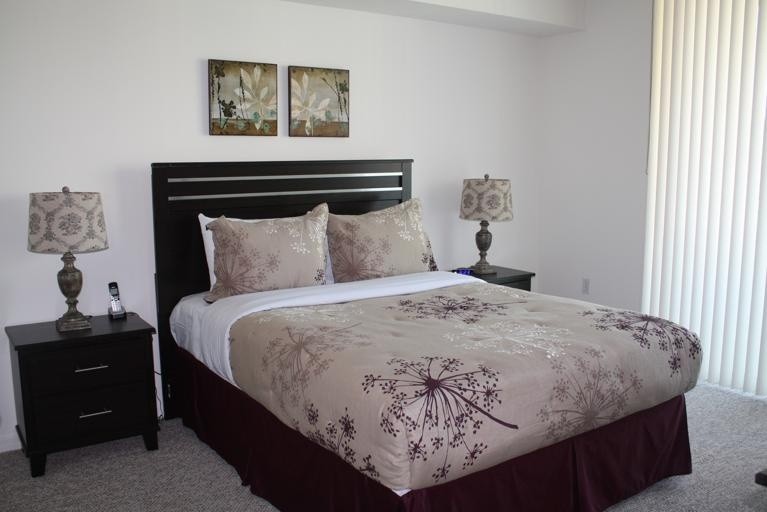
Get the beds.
[149,158,702,512]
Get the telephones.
[107,282,126,321]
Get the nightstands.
[449,263,535,292]
[4,311,157,478]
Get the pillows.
[197,197,439,304]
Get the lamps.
[458,173,514,275]
[26,186,108,333]
